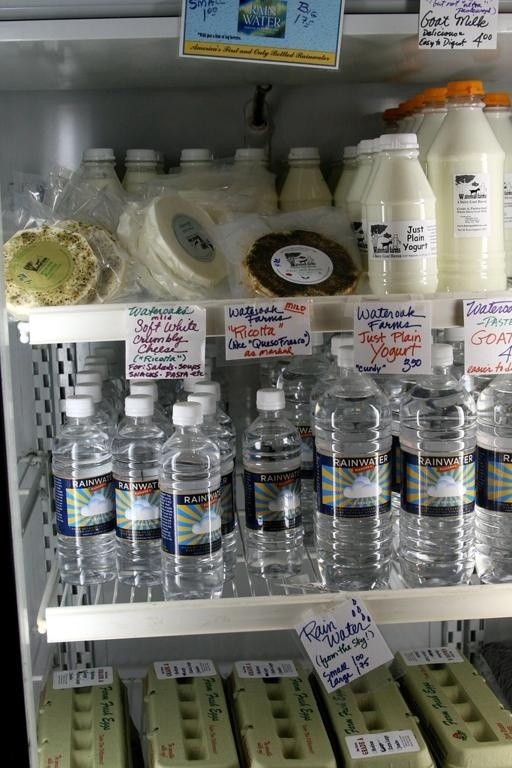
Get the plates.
[0,0,511,768]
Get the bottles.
[51,326,512,602]
[78,80,512,295]
